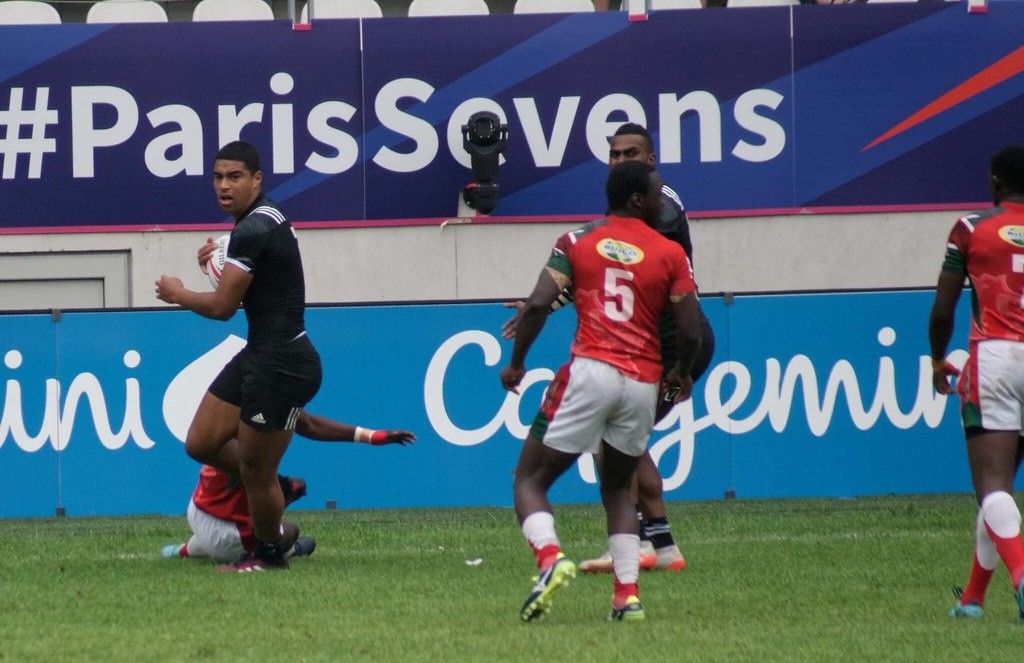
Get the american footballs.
[206,234,230,290]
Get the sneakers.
[603,595,645,621]
[520,551,576,623]
[1013,575,1024,624]
[647,545,685,572]
[162,543,177,557]
[579,541,658,574]
[283,478,305,508]
[218,544,289,573]
[282,536,315,560]
[947,601,984,617]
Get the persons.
[496,122,717,624]
[155,142,419,572]
[928,146,1024,626]
